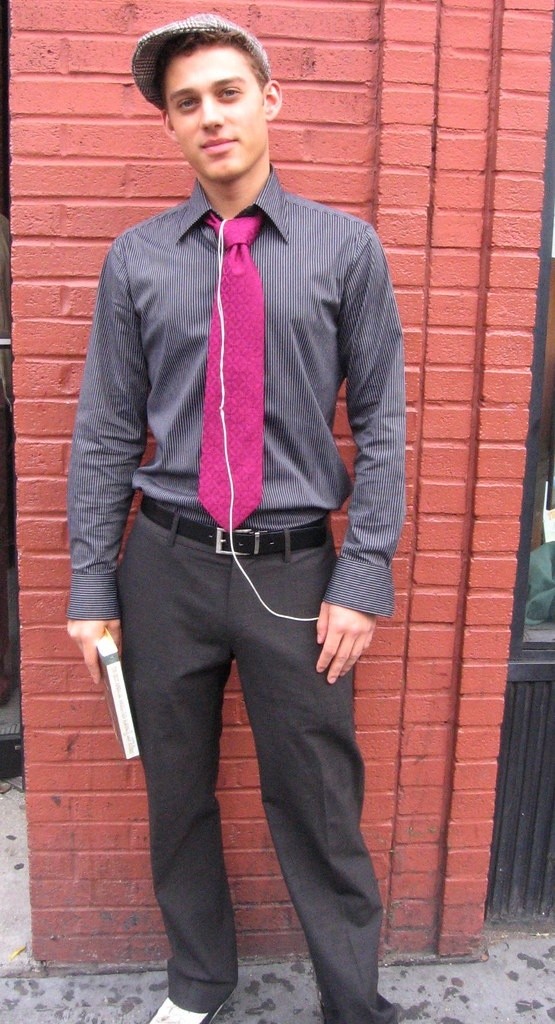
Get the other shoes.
[148,988,236,1024]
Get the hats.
[131,13,270,110]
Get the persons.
[67,12,403,1023]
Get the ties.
[199,208,265,532]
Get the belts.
[139,495,328,556]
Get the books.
[97,628,139,761]
